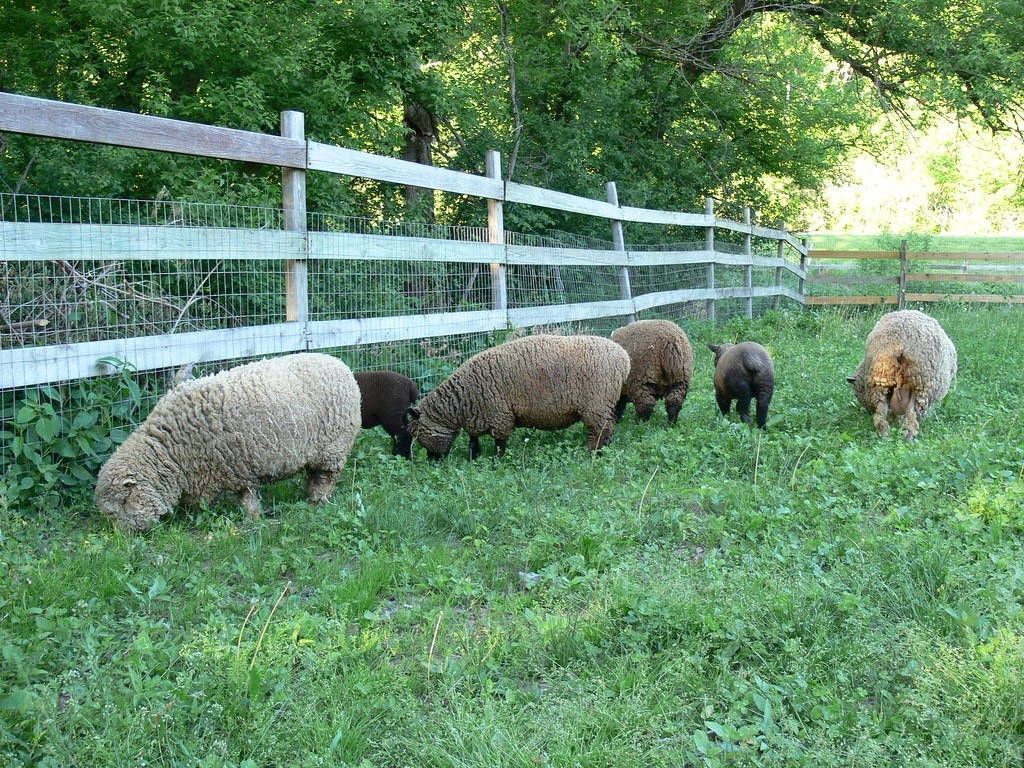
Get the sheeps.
[845,309,958,440]
[354,371,421,462]
[405,335,632,461]
[608,319,693,426]
[707,341,776,431]
[93,351,362,536]
